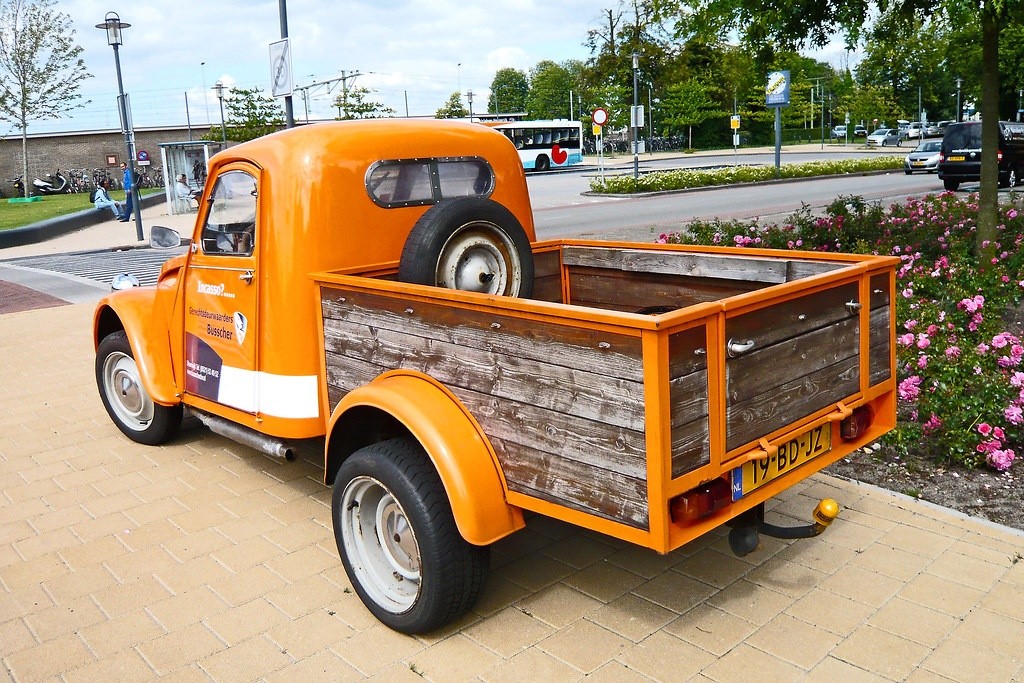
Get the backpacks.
[90,188,104,202]
[134,171,142,188]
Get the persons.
[177,173,201,209]
[582,135,685,155]
[119,162,139,222]
[526,137,534,145]
[192,159,206,188]
[95,179,125,220]
[518,139,524,148]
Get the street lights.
[952,78,965,122]
[200,61,210,124]
[95,10,148,241]
[828,92,832,144]
[464,89,476,122]
[457,62,461,93]
[210,80,230,149]
[1019,88,1024,110]
[631,51,644,180]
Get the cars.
[898,118,954,140]
[866,128,902,147]
[830,124,847,140]
[854,125,867,137]
[904,141,943,175]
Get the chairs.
[534,130,578,144]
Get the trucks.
[93,120,897,637]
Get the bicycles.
[62,167,165,192]
[582,134,689,158]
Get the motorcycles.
[30,168,72,195]
[4,174,25,199]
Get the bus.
[484,118,583,175]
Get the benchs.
[179,198,192,213]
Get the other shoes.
[117,216,122,219]
[119,219,129,222]
[132,218,136,221]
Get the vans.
[938,121,1024,192]
